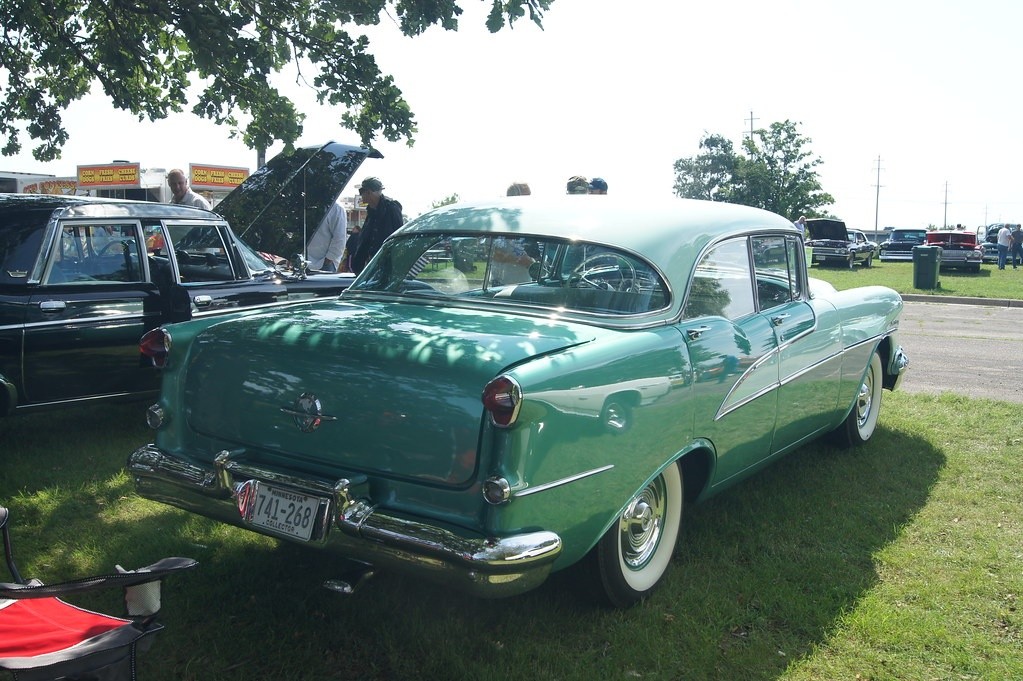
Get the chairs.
[0,507,199,681]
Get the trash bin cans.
[911,245,943,290]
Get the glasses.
[358,188,370,195]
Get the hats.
[566,176,589,193]
[587,177,608,191]
[354,177,385,192]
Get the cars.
[923,229,982,271]
[801,215,877,271]
[880,225,930,263]
[0,138,386,432]
[130,193,908,607]
[975,225,1023,265]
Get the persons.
[995,222,1015,269]
[1007,224,1023,269]
[565,176,588,195]
[359,176,404,269]
[305,198,347,272]
[586,178,609,195]
[166,168,213,212]
[482,182,543,288]
[345,225,362,272]
[93,225,110,254]
[953,224,962,231]
[794,215,806,238]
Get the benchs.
[492,286,666,312]
